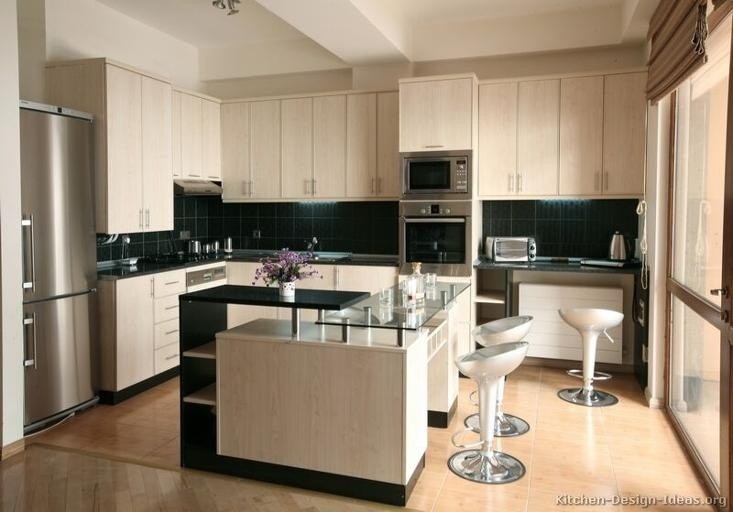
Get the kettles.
[609,229,633,262]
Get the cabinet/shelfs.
[173,86,220,182]
[96,274,152,393]
[220,94,280,205]
[396,72,476,155]
[345,84,402,202]
[226,262,397,331]
[471,269,506,327]
[179,281,431,504]
[477,74,559,201]
[152,268,186,378]
[559,64,647,201]
[44,56,173,236]
[280,89,344,203]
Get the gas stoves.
[144,253,226,285]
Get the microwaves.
[485,235,536,263]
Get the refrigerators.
[19,100,102,435]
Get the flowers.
[249,245,324,290]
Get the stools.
[461,313,534,439]
[445,340,529,486]
[553,305,624,410]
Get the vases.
[278,280,296,298]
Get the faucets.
[307,236,318,257]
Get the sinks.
[297,250,353,259]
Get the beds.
[314,273,473,508]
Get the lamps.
[212,1,245,16]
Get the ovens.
[398,149,473,277]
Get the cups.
[379,272,437,307]
[188,236,232,256]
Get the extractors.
[172,180,222,197]
